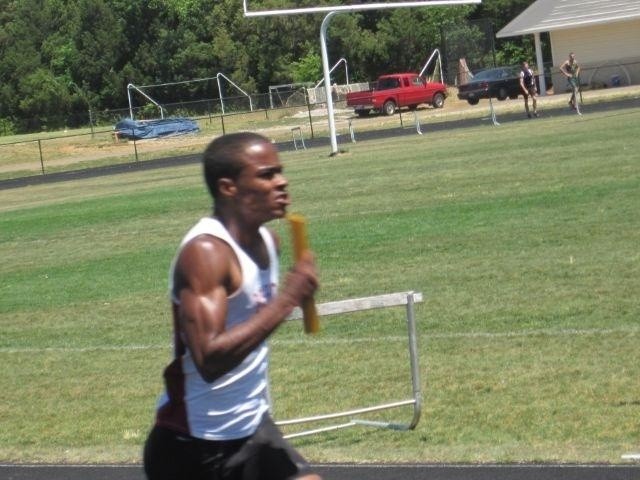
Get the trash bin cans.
[611,74,621,87]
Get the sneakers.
[527,113,540,118]
[568,102,582,116]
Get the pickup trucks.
[346,73,448,116]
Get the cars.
[457,67,551,104]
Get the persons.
[520,62,540,117]
[560,53,582,116]
[144,132,321,480]
